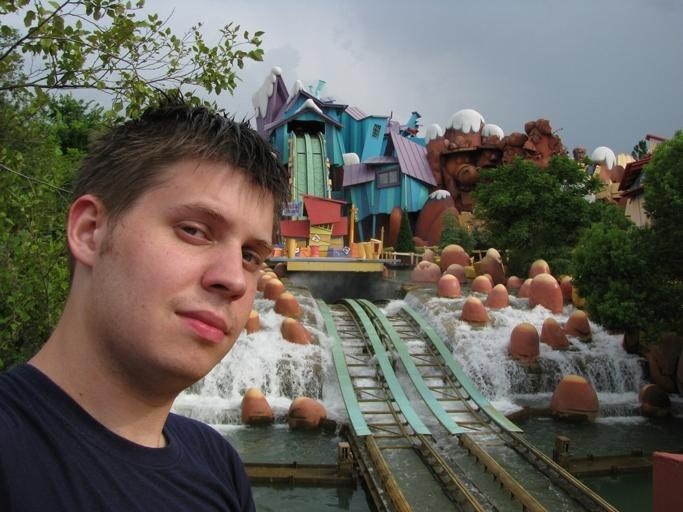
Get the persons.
[0,91,294,512]
